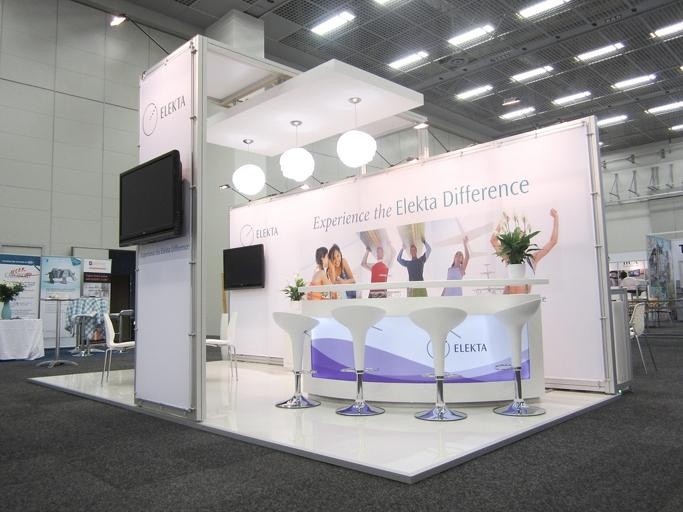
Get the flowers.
[0,280,23,302]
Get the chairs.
[629,302,659,374]
[205,310,238,380]
[100,314,135,383]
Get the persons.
[327,245,355,299]
[619,271,640,289]
[307,247,335,300]
[488,208,559,295]
[441,236,470,296]
[360,241,393,297]
[396,234,432,297]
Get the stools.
[407,306,469,421]
[271,311,322,408]
[329,303,386,415]
[109,309,133,353]
[493,298,545,416]
[70,311,98,357]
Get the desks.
[66,297,106,353]
[0,318,45,361]
[35,295,82,368]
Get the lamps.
[335,96,382,170]
[109,13,169,55]
[216,182,251,203]
[412,122,450,154]
[279,118,315,183]
[232,138,266,196]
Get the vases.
[1,300,12,320]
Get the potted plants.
[280,275,308,311]
[491,211,539,280]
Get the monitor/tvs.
[222,243,266,289]
[118,149,185,247]
